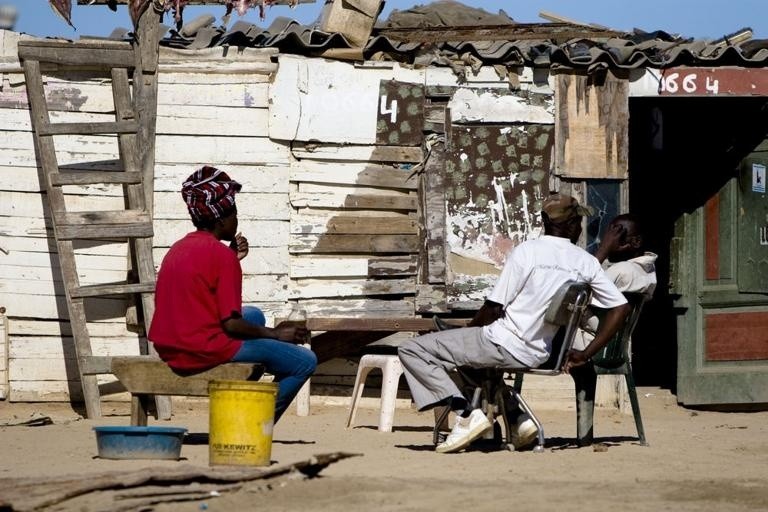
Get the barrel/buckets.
[207,378,279,467]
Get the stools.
[342,347,443,432]
[112,352,264,431]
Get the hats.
[540,193,596,226]
[181,166,241,225]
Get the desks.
[273,312,478,420]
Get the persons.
[397,193,632,453]
[431,213,657,369]
[147,166,318,426]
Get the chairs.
[429,290,587,452]
[570,289,651,445]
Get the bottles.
[287,308,308,330]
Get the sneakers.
[434,408,494,453]
[512,414,539,450]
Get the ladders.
[20,42,171,421]
